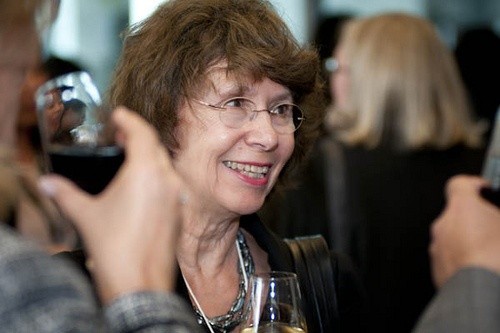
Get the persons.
[98,0,338,333]
[1,0,200,333]
[54,98,86,145]
[324,9,487,333]
[416,175,500,333]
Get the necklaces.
[191,229,256,333]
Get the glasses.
[191,95,306,134]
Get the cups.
[34,71,125,196]
[478,108,500,208]
[237,272,307,333]
